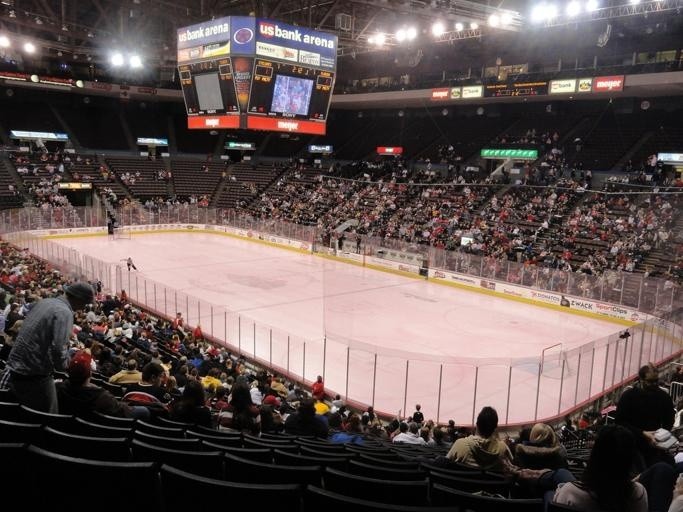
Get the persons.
[0,237,250,365]
[0,365,682,512]
[6,148,294,237]
[295,128,579,297]
[578,150,681,318]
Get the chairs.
[548,100,682,324]
[227,347,682,511]
[0,234,225,511]
[0,93,228,227]
[228,106,548,286]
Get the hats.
[362,412,369,423]
[64,282,94,303]
[68,348,91,376]
[264,395,281,407]
